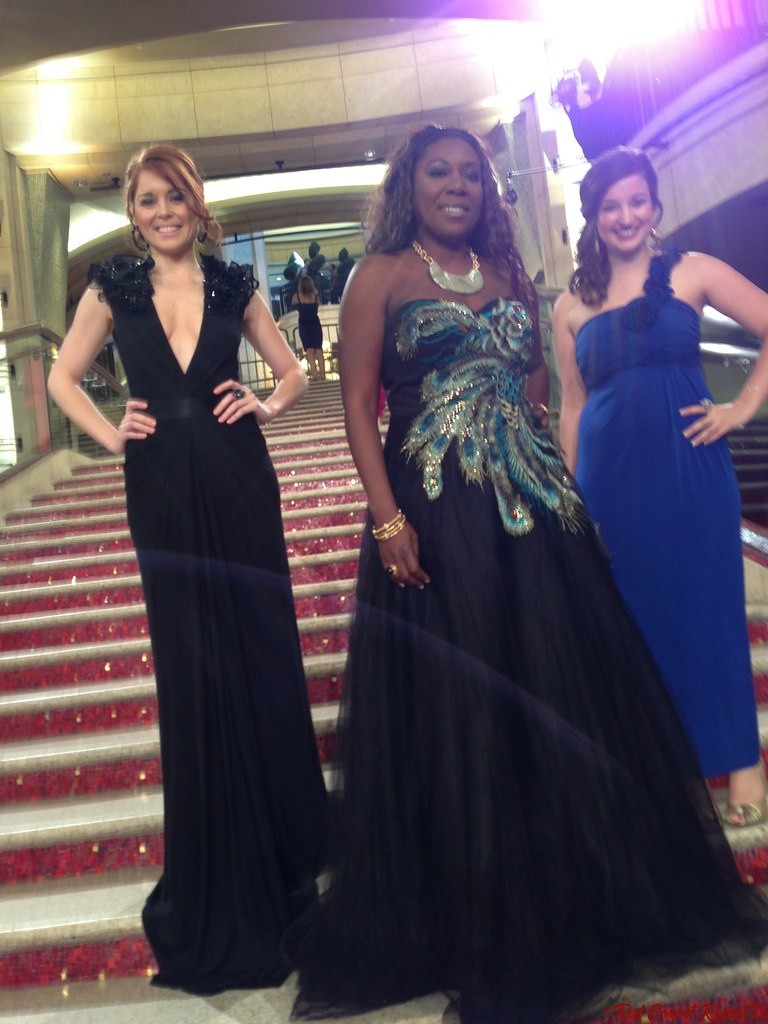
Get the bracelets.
[371,508,407,542]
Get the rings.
[700,397,714,412]
[233,389,246,399]
[386,563,399,578]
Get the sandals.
[723,752,767,826]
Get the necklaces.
[411,238,484,295]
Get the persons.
[333,120,597,1023]
[552,143,768,829]
[329,264,338,305]
[46,143,340,999]
[296,259,311,278]
[292,276,327,382]
